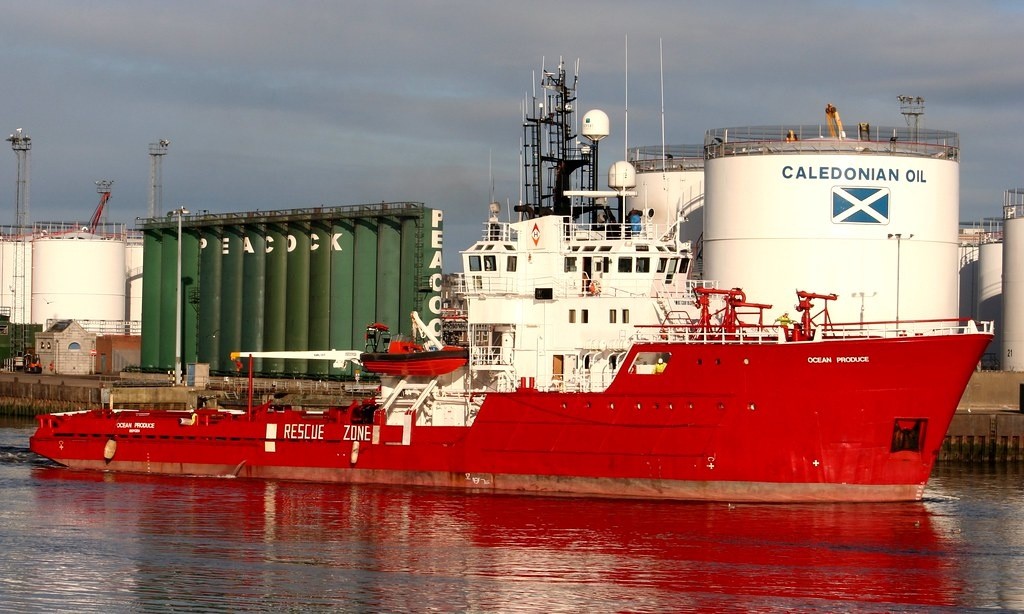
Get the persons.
[775,312,793,325]
[189,409,198,426]
[651,358,667,374]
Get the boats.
[29,35,995,502]
[361,324,468,378]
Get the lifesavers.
[590,281,602,294]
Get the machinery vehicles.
[67,180,114,234]
[26,353,42,375]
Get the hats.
[658,358,663,364]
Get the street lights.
[145,137,170,221]
[5,132,30,359]
[167,206,190,385]
